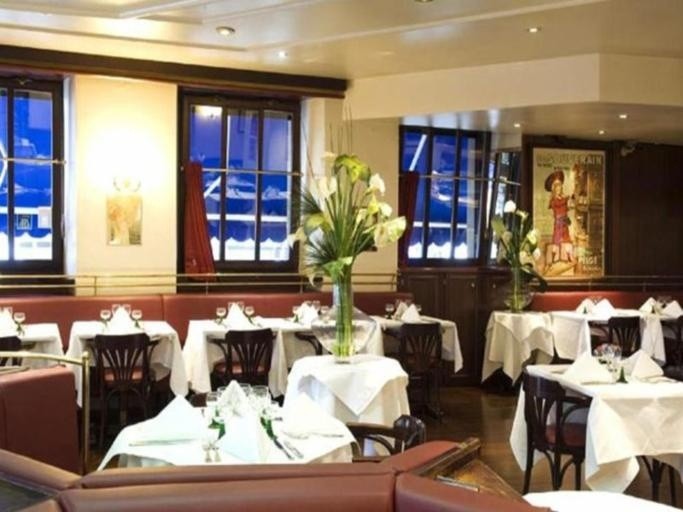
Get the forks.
[202,440,220,463]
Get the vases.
[495,265,535,312]
[326,267,355,361]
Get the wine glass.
[384,303,394,320]
[244,306,255,325]
[13,311,26,333]
[215,306,226,326]
[292,305,301,323]
[131,309,142,328]
[99,309,111,329]
[594,344,622,373]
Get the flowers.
[270,101,404,357]
[487,195,552,313]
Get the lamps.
[101,173,150,251]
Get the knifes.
[274,439,304,460]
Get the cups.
[111,304,130,316]
[205,383,270,407]
[301,300,320,313]
[2,307,12,317]
[394,299,415,310]
[226,301,244,314]
[319,305,329,314]
[414,304,422,311]
